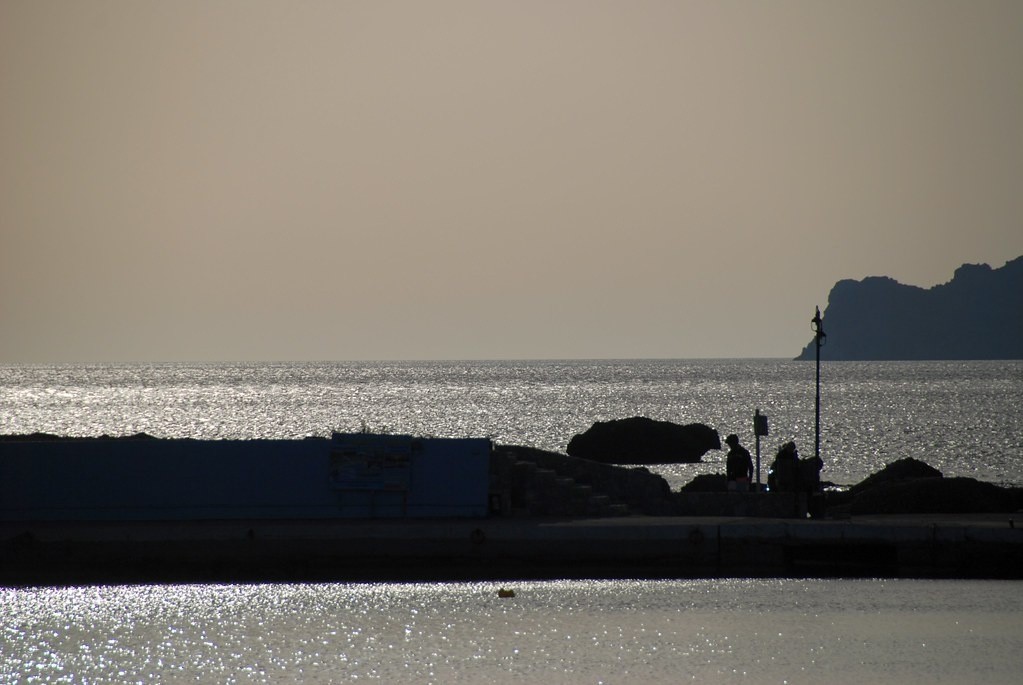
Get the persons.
[725,433,754,492]
[775,441,833,492]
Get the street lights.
[810,305,828,456]
